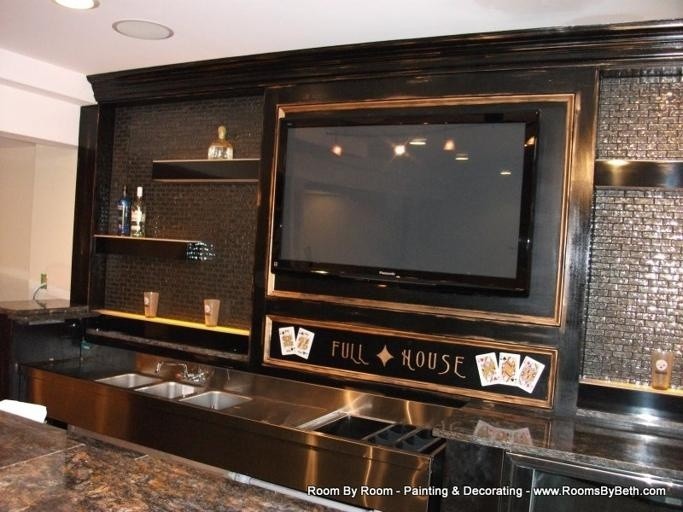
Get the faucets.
[154,357,195,382]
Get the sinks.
[90,369,166,390]
[178,391,252,411]
[131,380,205,401]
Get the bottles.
[207,125,233,160]
[130,186,147,237]
[117,184,131,236]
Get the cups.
[143,291,160,317]
[650,351,674,390]
[204,298,220,327]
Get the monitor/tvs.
[270,107,540,298]
